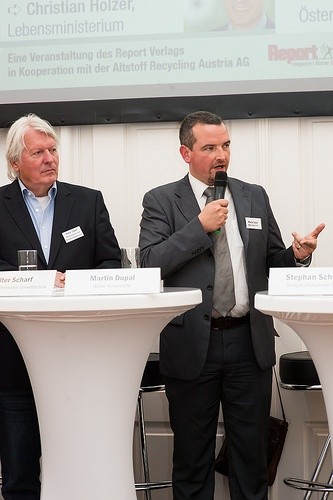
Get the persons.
[0,114,121,500]
[134,111,326,500]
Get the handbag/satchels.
[215,413,288,486]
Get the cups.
[121,247,141,268]
[17,249,38,271]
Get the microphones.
[211,171,228,236]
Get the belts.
[210,314,250,331]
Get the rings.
[297,245,302,249]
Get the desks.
[254,290,333,466]
[0,287,203,500]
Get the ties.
[204,186,236,317]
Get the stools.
[279,351,333,500]
[134,352,173,500]
